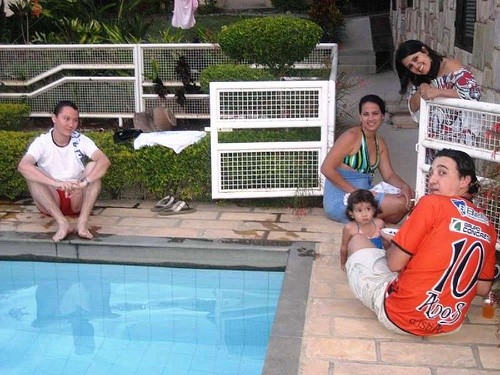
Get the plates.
[380,227,399,236]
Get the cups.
[482,291,497,318]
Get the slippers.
[159,201,196,216]
[150,196,182,212]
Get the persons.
[395,40,500,212]
[345,148,497,338]
[321,94,416,224]
[18,100,111,243]
[340,189,391,272]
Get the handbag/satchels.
[112,127,144,149]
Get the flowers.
[7,1,43,17]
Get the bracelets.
[83,178,90,185]
